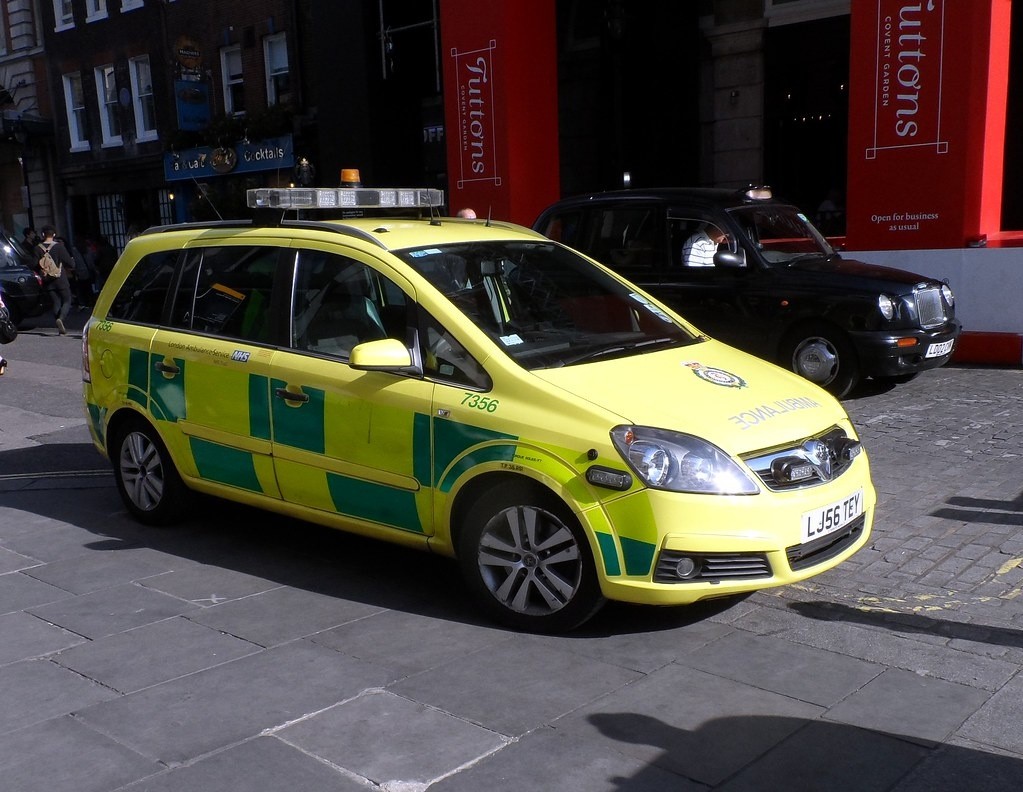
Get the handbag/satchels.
[0,308,18,344]
[88,269,97,284]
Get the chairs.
[296,287,388,360]
[673,228,698,261]
[621,223,651,248]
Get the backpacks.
[37,243,62,278]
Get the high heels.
[0,359,8,375]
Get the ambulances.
[79,186,882,637]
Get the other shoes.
[56,319,66,333]
[59,332,68,337]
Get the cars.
[0,224,49,328]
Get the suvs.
[505,188,956,408]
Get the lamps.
[167,190,175,201]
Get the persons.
[20,225,119,334]
[683,222,727,267]
